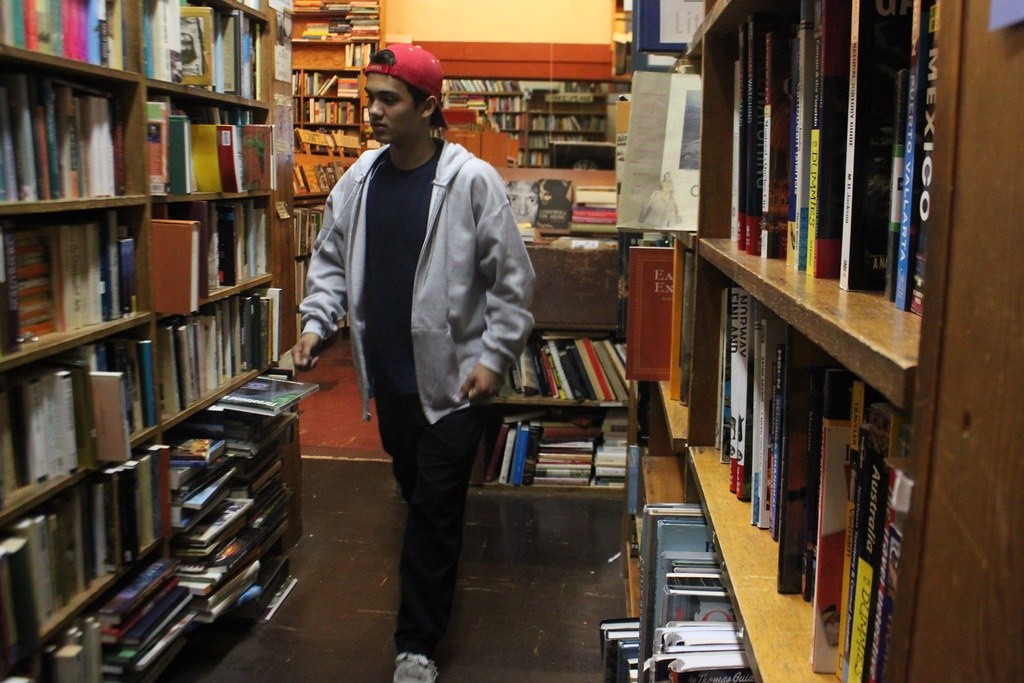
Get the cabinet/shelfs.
[2,0,1024,681]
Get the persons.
[296,44,535,683]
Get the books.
[150,218,200,316]
[143,0,277,195]
[0,287,298,682]
[635,0,706,52]
[211,205,265,287]
[293,0,630,256]
[598,503,756,683]
[680,251,696,408]
[168,200,210,298]
[627,442,641,512]
[210,373,319,417]
[617,70,703,233]
[669,239,682,403]
[714,239,918,682]
[0,0,161,354]
[625,246,676,381]
[731,0,941,318]
[469,330,629,490]
[636,380,649,445]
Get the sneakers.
[393,651,437,683]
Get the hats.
[366,43,450,129]
